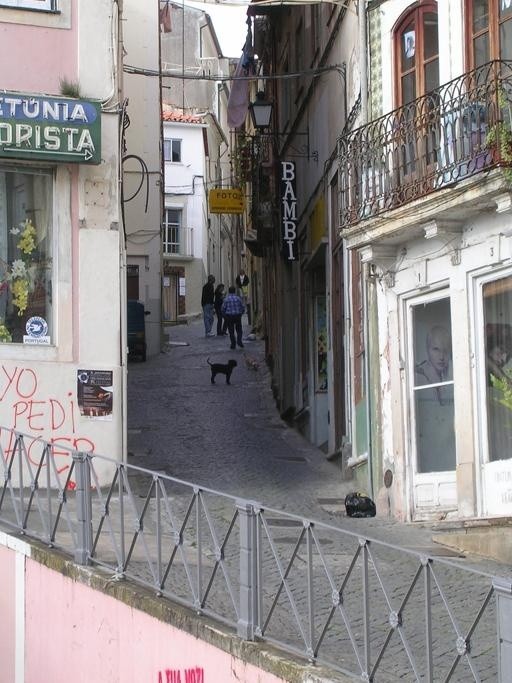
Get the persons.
[199,273,216,336]
[220,285,245,349]
[214,282,228,335]
[235,268,250,300]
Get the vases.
[13,288,45,335]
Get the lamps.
[247,89,310,161]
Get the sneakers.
[205,332,216,337]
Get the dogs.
[207,358,238,385]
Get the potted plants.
[483,125,512,166]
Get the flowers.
[4,218,52,322]
